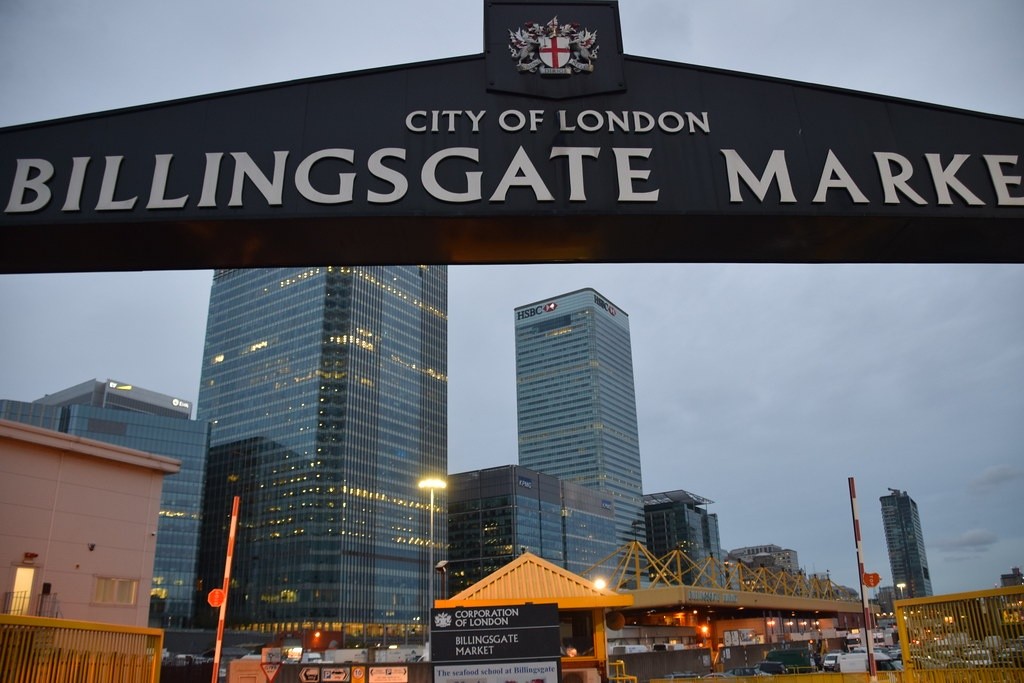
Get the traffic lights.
[312,630,323,649]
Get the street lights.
[416,477,455,645]
[897,582,907,599]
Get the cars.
[662,627,1024,679]
